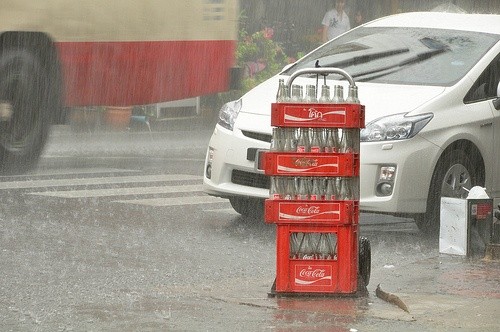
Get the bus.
[0,0,245,170]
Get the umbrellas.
[430,3,468,14]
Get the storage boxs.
[276,223,360,293]
[264,152,360,177]
[264,199,358,224]
[271,103,365,128]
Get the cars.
[202,11,500,236]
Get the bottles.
[269,127,354,154]
[289,232,336,260]
[269,175,355,200]
[275,78,360,104]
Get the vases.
[70,107,99,134]
[103,106,133,132]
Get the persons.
[355,10,368,26]
[322,0,351,44]
